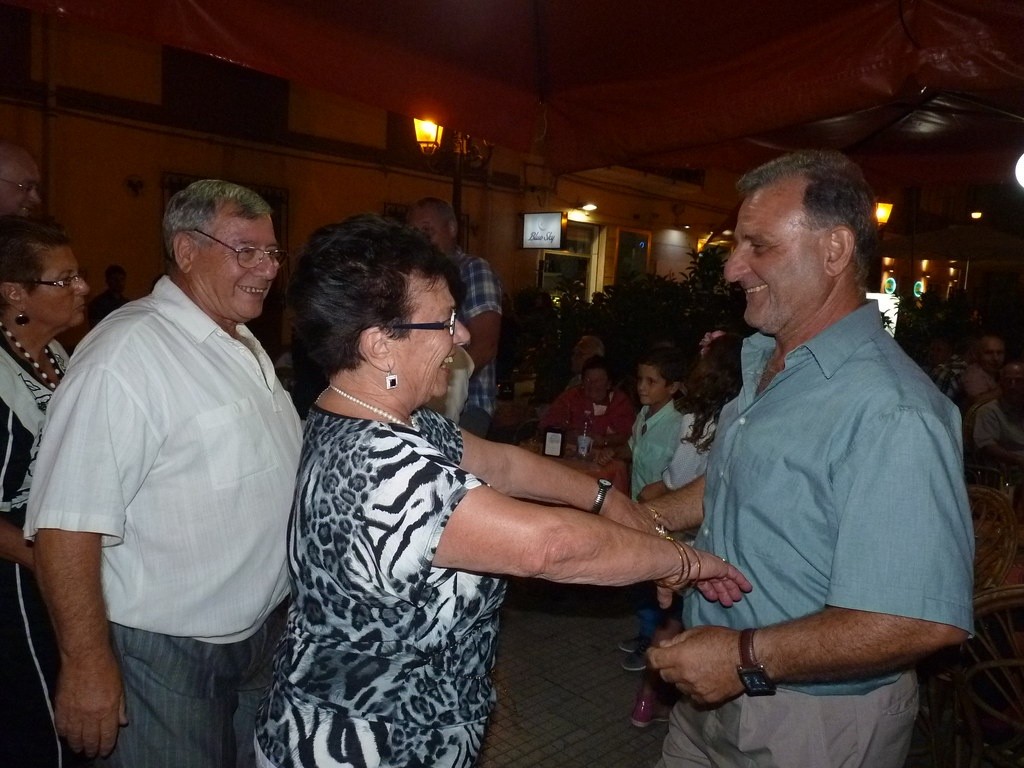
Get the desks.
[520,442,630,498]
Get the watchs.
[737,627,776,694]
[591,478,612,515]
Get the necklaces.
[0,321,62,390]
[330,385,405,425]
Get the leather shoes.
[629,690,672,727]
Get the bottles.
[576,411,593,458]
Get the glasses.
[388,309,459,335]
[191,227,289,272]
[0,178,44,194]
[11,269,85,288]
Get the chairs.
[912,389,1024,768]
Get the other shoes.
[620,638,650,671]
[617,635,648,652]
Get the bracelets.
[653,536,700,592]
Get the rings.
[648,508,661,521]
[655,522,667,535]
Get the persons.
[0,213,90,768]
[924,328,1024,484]
[595,342,685,676]
[539,336,638,450]
[253,214,753,768]
[0,141,42,217]
[408,196,502,442]
[87,264,129,329]
[630,331,744,727]
[647,149,975,768]
[24,179,303,768]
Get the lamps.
[675,223,692,229]
[576,202,598,212]
